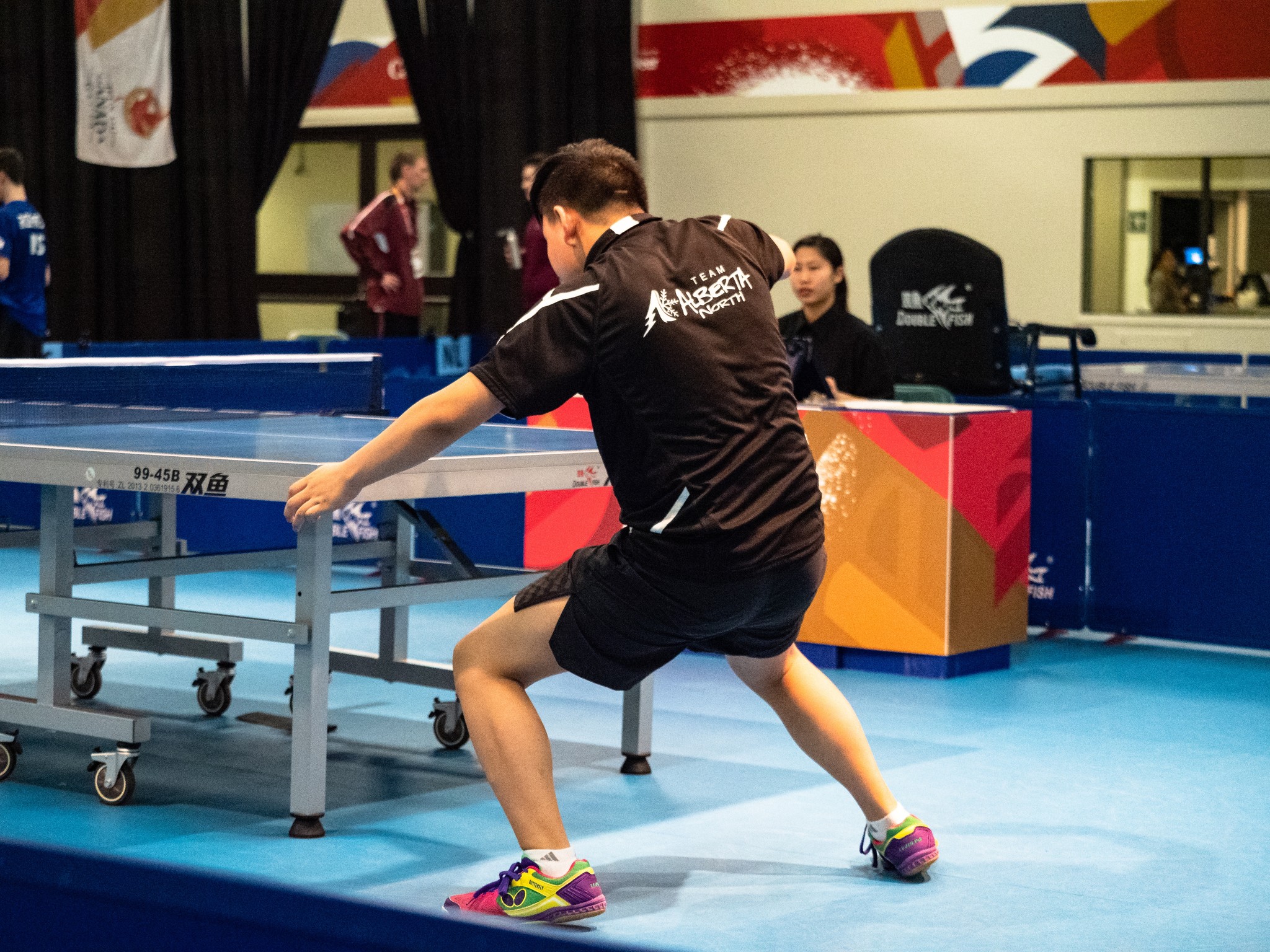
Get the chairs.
[865,222,1097,398]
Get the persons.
[778,234,895,399]
[282,139,938,925]
[1146,249,1188,314]
[341,150,430,337]
[0,149,52,357]
[503,154,560,321]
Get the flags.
[74,0,178,169]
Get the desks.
[0,345,665,840]
[522,393,1036,681]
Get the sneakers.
[442,856,608,924]
[860,811,939,876]
